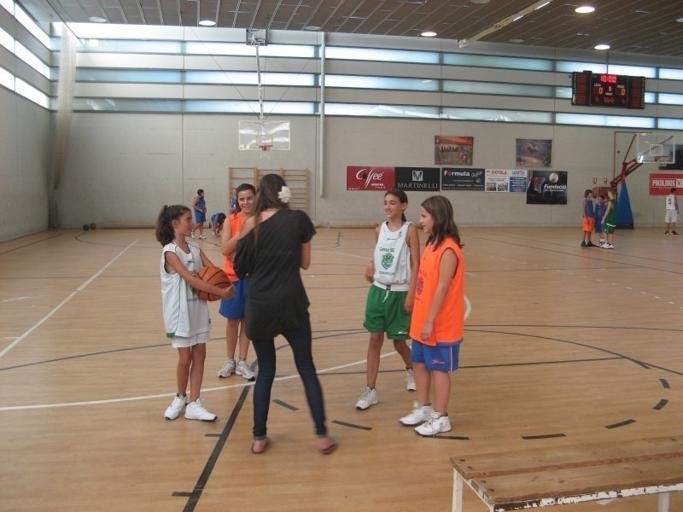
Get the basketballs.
[90,223,96,229]
[548,173,558,184]
[191,267,231,302]
[82,225,88,231]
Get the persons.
[601,188,619,249]
[233,174,337,454]
[211,212,224,236]
[399,196,465,437]
[191,188,208,239]
[665,187,680,236]
[581,189,595,248]
[595,194,607,248]
[354,188,420,409]
[155,205,234,421]
[217,183,257,380]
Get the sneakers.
[663,231,669,235]
[413,411,450,437]
[405,366,417,391]
[234,358,256,381]
[191,231,197,238]
[217,359,236,378]
[600,243,611,248]
[595,239,604,246]
[580,240,587,247]
[164,392,187,420]
[587,240,595,247]
[199,235,206,240]
[355,386,379,410]
[184,396,217,421]
[670,231,679,235]
[398,404,435,425]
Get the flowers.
[278,186,292,203]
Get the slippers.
[319,439,336,455]
[251,436,271,454]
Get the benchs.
[449,434,683,512]
